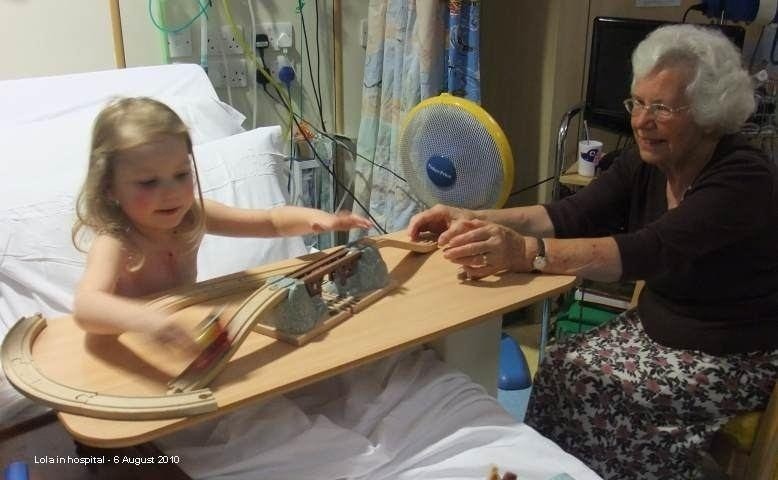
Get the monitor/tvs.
[584,16,745,133]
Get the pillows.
[0,127,308,434]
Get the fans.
[400,92,514,220]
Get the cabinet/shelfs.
[539,102,645,372]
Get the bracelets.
[531,236,549,274]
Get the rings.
[484,256,488,265]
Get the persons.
[407,22,778,479]
[69,95,374,351]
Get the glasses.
[624,99,690,119]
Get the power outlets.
[198,23,294,95]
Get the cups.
[577,140,604,177]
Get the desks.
[34,222,577,451]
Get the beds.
[0,59,610,479]
